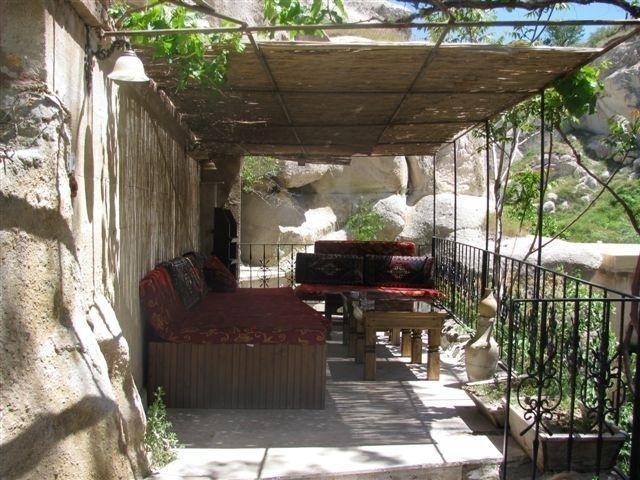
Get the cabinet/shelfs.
[213,207,239,280]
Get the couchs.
[294,240,442,320]
[139,252,330,409]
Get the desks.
[339,291,448,381]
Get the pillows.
[203,255,237,293]
[295,253,436,289]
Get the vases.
[465,288,502,382]
